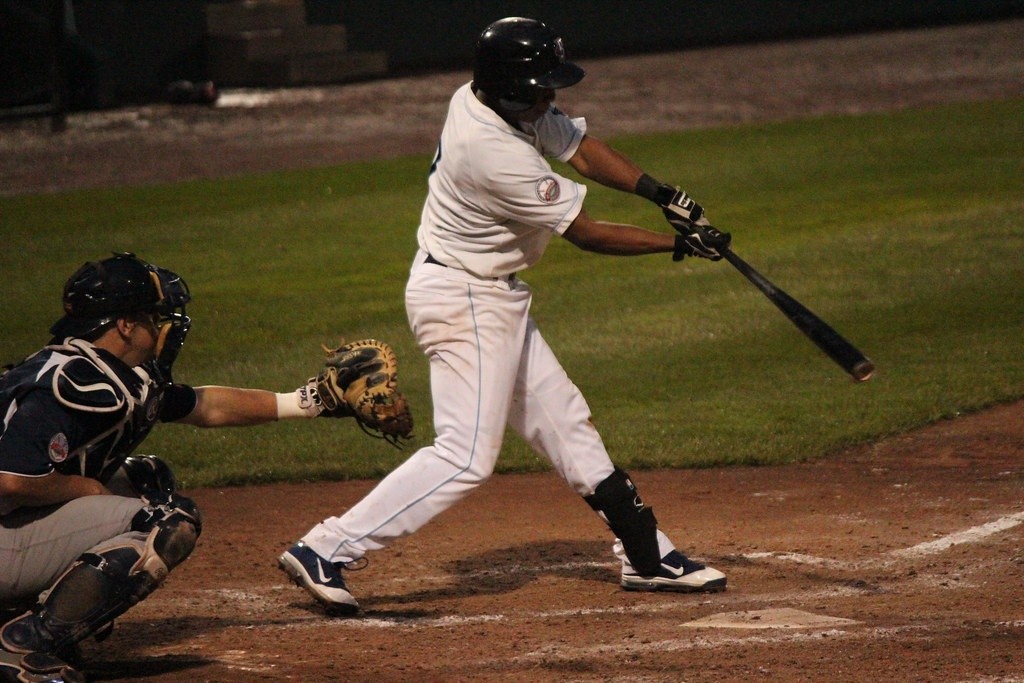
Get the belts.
[424,254,516,281]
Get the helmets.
[473,16,585,102]
[48,256,157,338]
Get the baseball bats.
[693,222,878,385]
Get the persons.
[279,14,735,609]
[0,249,413,683]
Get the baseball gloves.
[308,335,419,452]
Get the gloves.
[673,225,731,262]
[656,182,710,237]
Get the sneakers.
[277,539,369,615]
[619,547,728,593]
[1,627,76,683]
